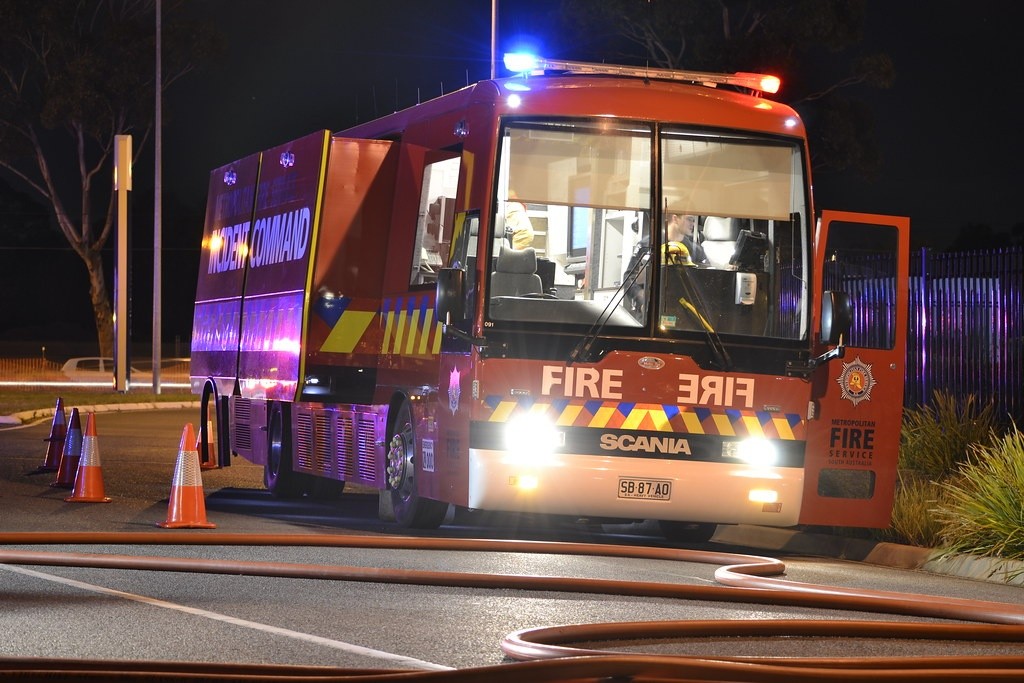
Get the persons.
[623,212,711,312]
[504,189,534,250]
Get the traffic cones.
[61,412,112,504]
[155,423,215,529]
[50,407,83,489]
[192,402,221,469]
[38,397,69,471]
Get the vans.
[61,356,143,376]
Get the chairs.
[488,247,543,297]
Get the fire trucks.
[189,54,906,548]
